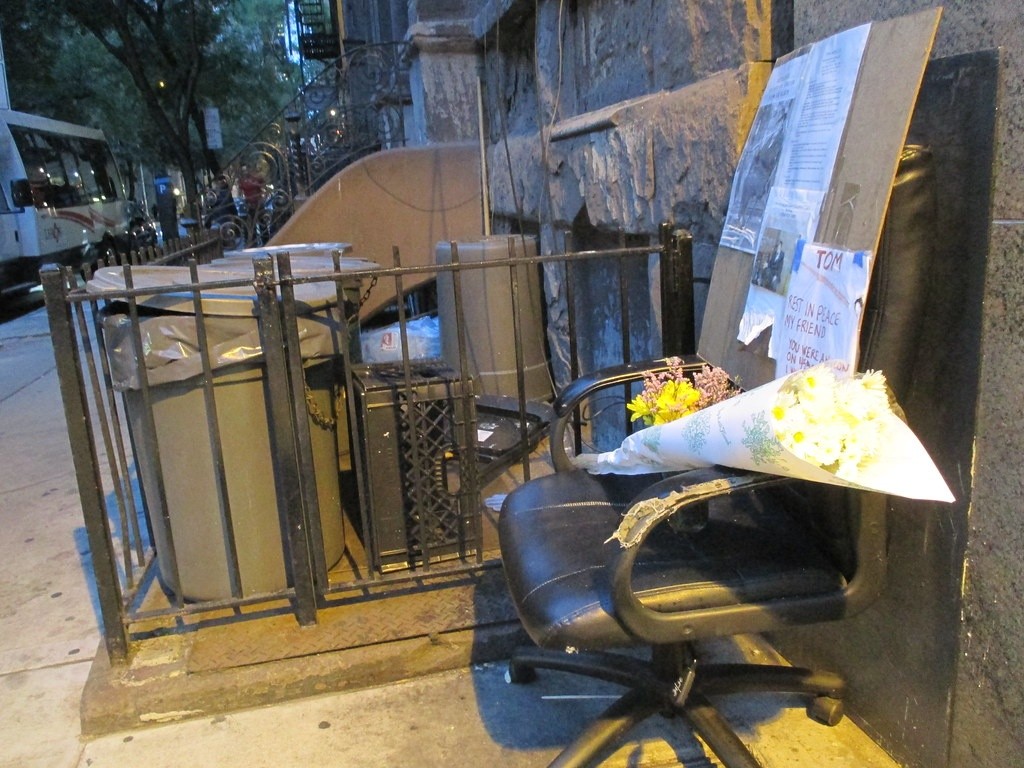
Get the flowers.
[773,369,890,478]
[627,354,745,427]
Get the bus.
[0,108,131,308]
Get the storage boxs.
[352,355,483,576]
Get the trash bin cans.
[86,241,379,604]
[344,357,479,575]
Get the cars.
[130,201,157,253]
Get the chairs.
[498,130,937,768]
